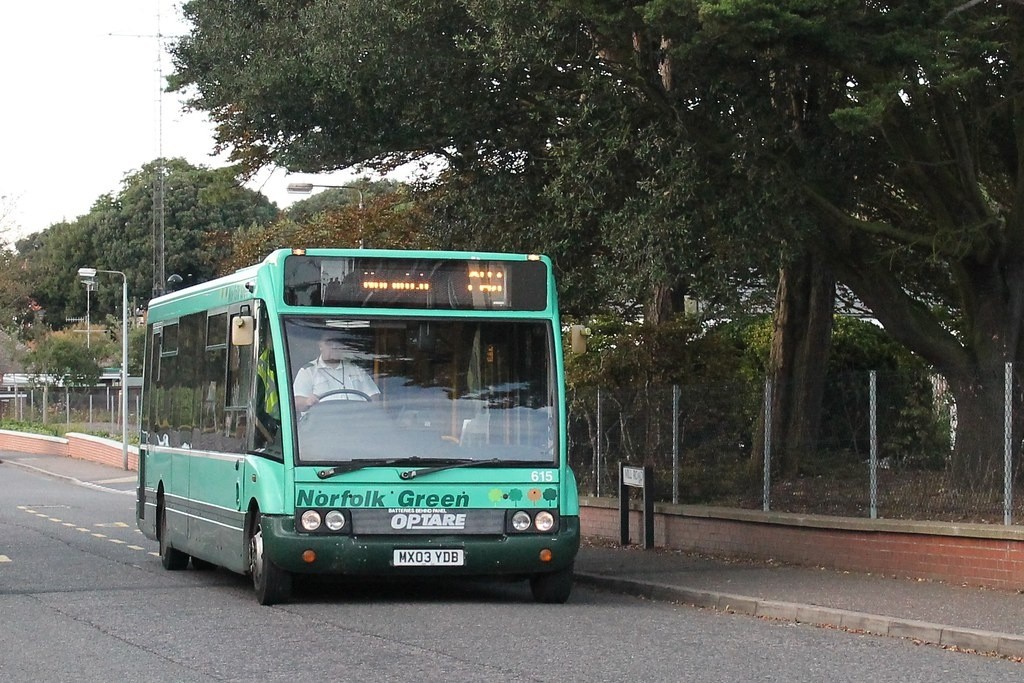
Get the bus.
[133,248,580,607]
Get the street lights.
[77,267,129,472]
[284,182,364,249]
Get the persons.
[292,326,381,421]
